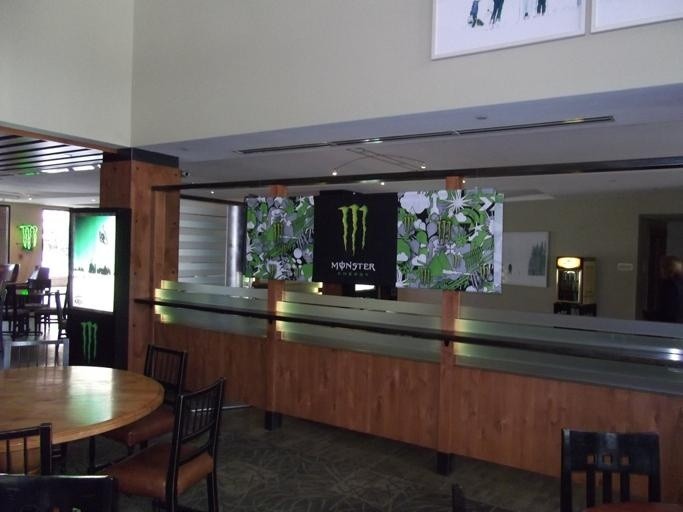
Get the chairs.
[0,470,114,511]
[562,428,660,512]
[87,342,186,467]
[451,484,512,512]
[0,421,53,476]
[101,376,229,512]
[0,262,68,358]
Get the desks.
[555,301,599,317]
[0,364,164,475]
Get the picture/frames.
[502,229,550,288]
[589,0,683,34]
[429,0,585,62]
